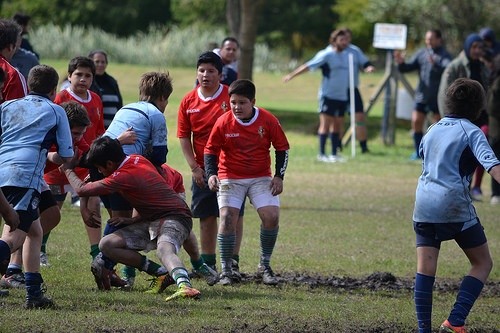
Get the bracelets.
[191,166,199,172]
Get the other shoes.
[73,200,81,207]
[329,154,347,162]
[471,189,484,201]
[317,153,331,163]
[100,202,104,208]
[490,196,500,207]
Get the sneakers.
[197,263,218,286]
[122,275,135,290]
[24,296,60,310]
[40,252,48,267]
[188,264,218,279]
[0,289,9,298]
[91,251,112,291]
[143,272,175,294]
[257,265,279,285]
[165,284,201,301]
[2,273,26,290]
[110,269,126,288]
[219,269,233,286]
[231,265,242,283]
[440,319,466,333]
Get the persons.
[283,27,375,163]
[177,53,245,279]
[393,26,500,204]
[0,14,218,308]
[413,78,500,333]
[204,80,290,285]
[198,38,237,85]
